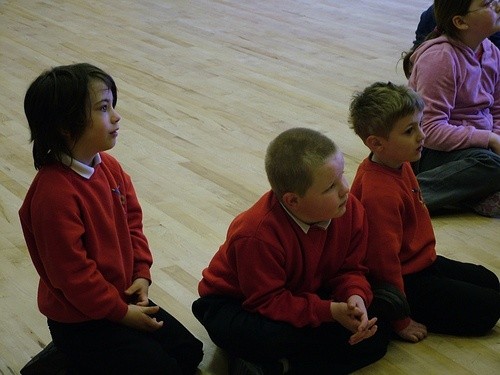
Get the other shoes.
[227,357,264,375]
[20,341,64,375]
[471,192,500,217]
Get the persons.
[396,0,500,218]
[348,81,500,343]
[18,62,204,375]
[191,128,389,375]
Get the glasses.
[468,0,500,13]
